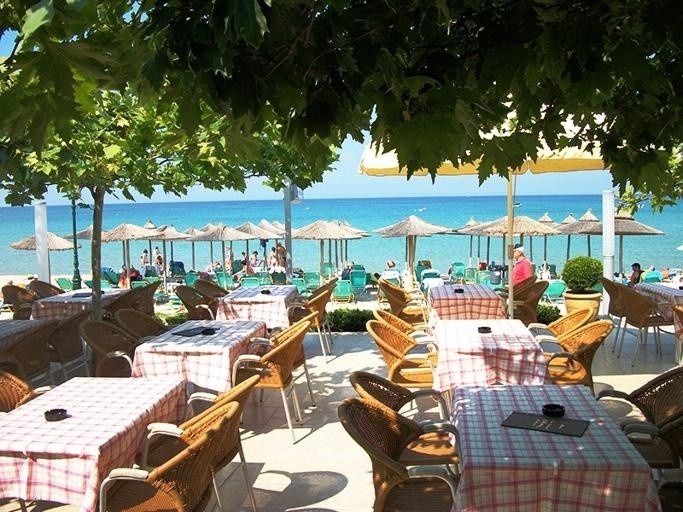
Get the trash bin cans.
[487,264,514,285]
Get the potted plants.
[560,254,605,318]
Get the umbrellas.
[360,93,679,319]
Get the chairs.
[80,308,318,448]
[2,372,261,512]
[366,308,615,399]
[173,274,340,367]
[599,276,683,367]
[335,366,683,512]
[378,274,552,323]
[0,260,160,381]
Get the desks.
[450,383,662,511]
[424,318,548,385]
[126,318,269,392]
[634,280,683,324]
[424,281,509,322]
[216,283,300,327]
[2,317,62,365]
[1,377,195,511]
[27,286,129,322]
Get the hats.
[514,244,525,252]
